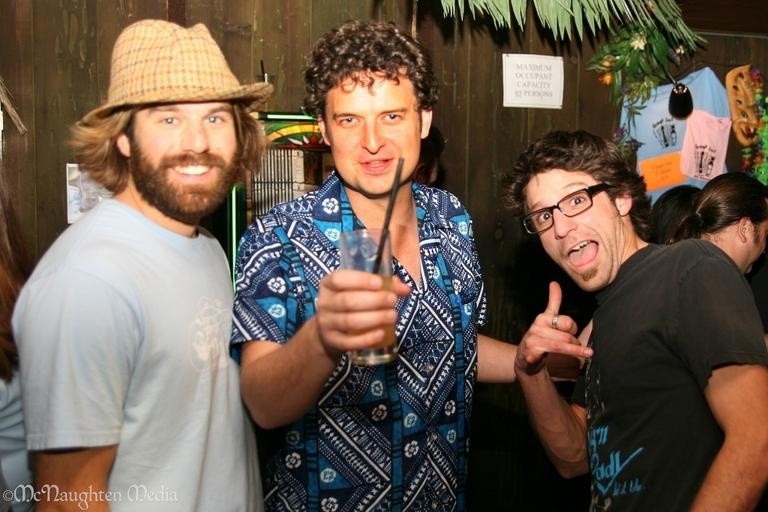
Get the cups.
[338,229,396,366]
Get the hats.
[80,19,275,125]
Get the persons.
[7,17,279,512]
[226,17,592,512]
[0,162,37,512]
[656,171,766,282]
[492,124,768,512]
[644,182,701,248]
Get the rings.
[551,313,559,330]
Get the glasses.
[523,182,611,234]
